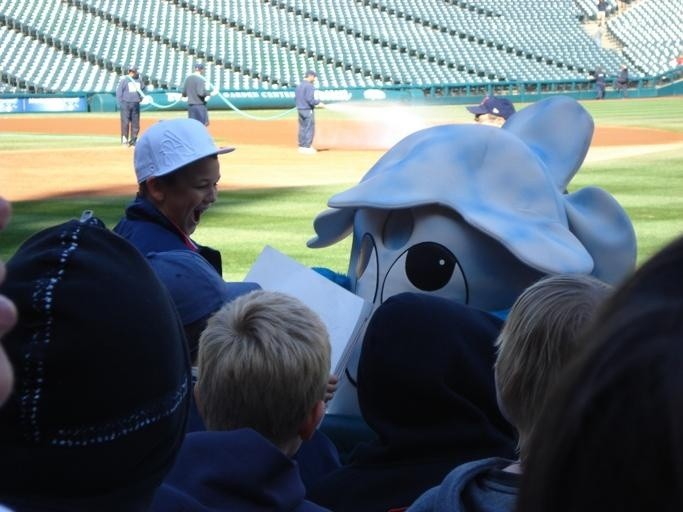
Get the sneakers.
[122,137,135,145]
[299,147,316,154]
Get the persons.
[522,233,682,512]
[1,201,190,511]
[295,71,324,152]
[182,65,211,127]
[145,255,339,406]
[113,117,235,279]
[116,67,144,146]
[306,292,518,511]
[407,275,616,511]
[591,66,629,99]
[173,291,331,511]
[466,95,515,128]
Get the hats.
[133,117,235,186]
[128,66,138,72]
[195,64,203,70]
[306,71,317,75]
[465,97,515,120]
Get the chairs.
[0,0,683,97]
